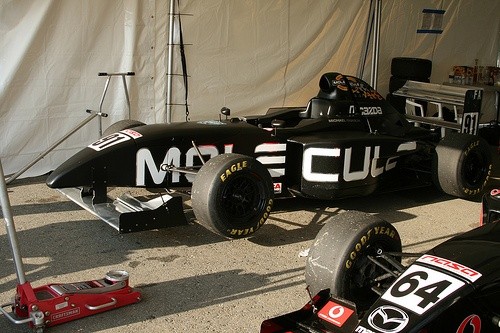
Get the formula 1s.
[257,182,500,333]
[45,70,493,239]
